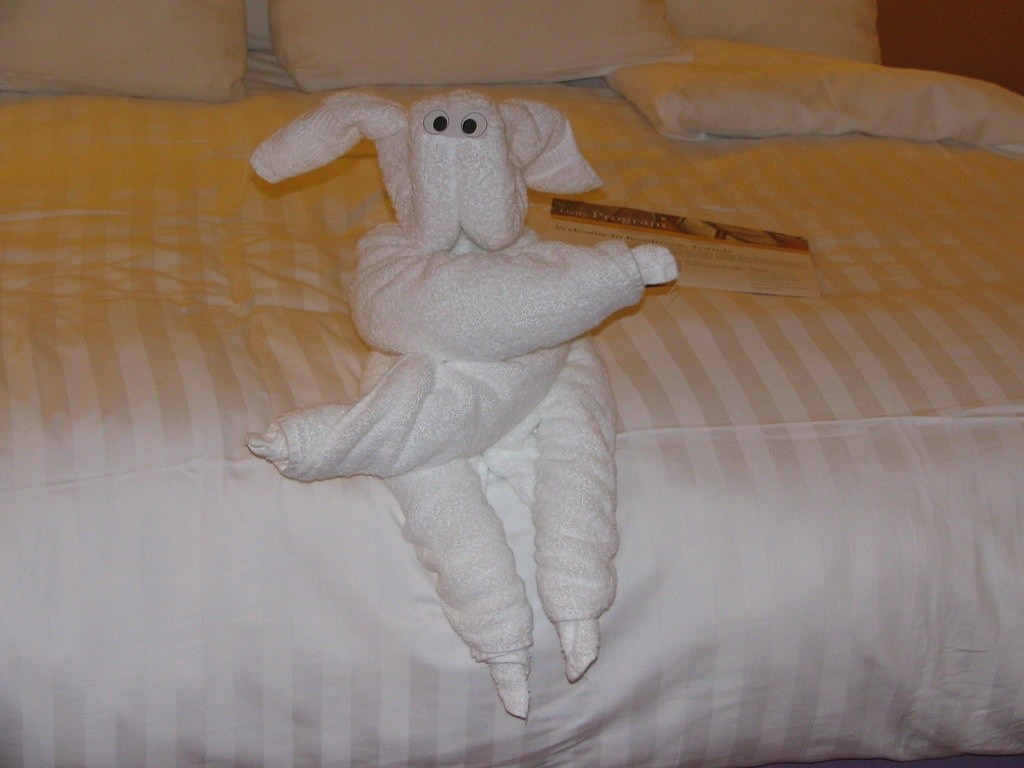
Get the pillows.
[267,0,693,96]
[0,0,250,101]
[662,1,881,66]
[602,38,1023,155]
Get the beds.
[0,0,1024,767]
